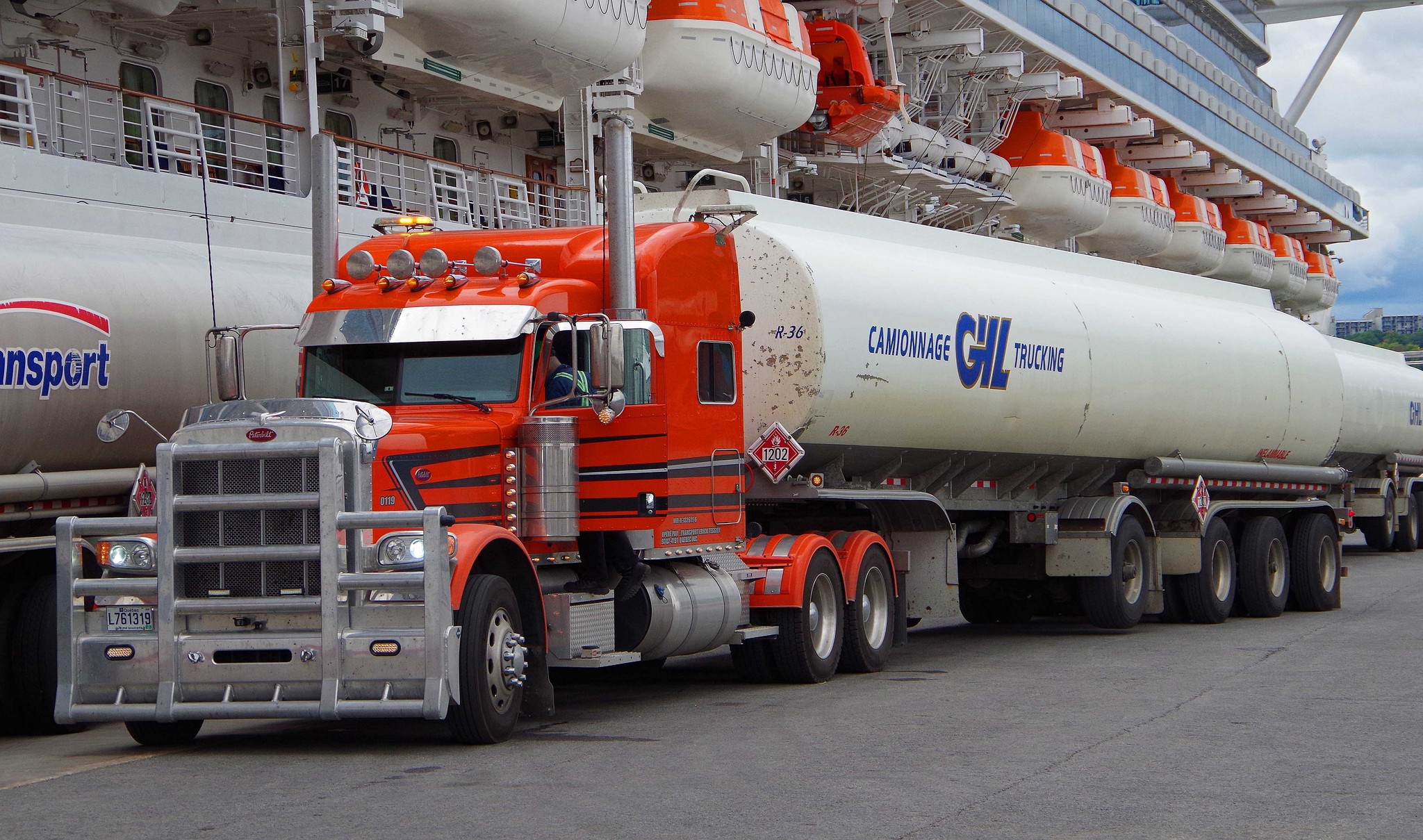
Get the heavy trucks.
[53,111,1354,743]
[1319,333,1423,552]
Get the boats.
[953,111,1112,241]
[1075,148,1175,262]
[1252,219,1310,303]
[628,1,821,163]
[358,0,654,112]
[1287,239,1342,315]
[1199,203,1276,287]
[0,0,1421,553]
[803,15,908,147]
[1138,177,1227,275]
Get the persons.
[546,351,651,602]
